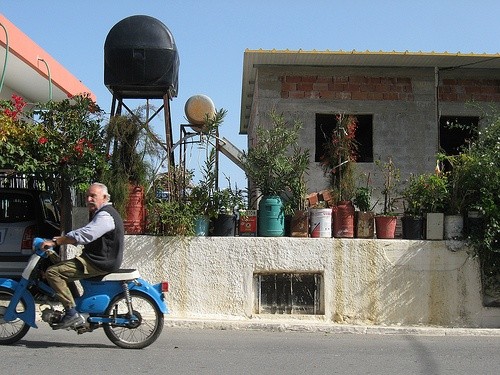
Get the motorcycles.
[0,238,171,350]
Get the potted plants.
[115,115,483,242]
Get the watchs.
[52,239,58,247]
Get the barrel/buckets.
[185,95,217,132]
[104,15,181,87]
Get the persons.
[40,183,125,331]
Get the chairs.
[7,202,28,218]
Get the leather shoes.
[52,313,85,330]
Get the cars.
[0,188,62,269]
